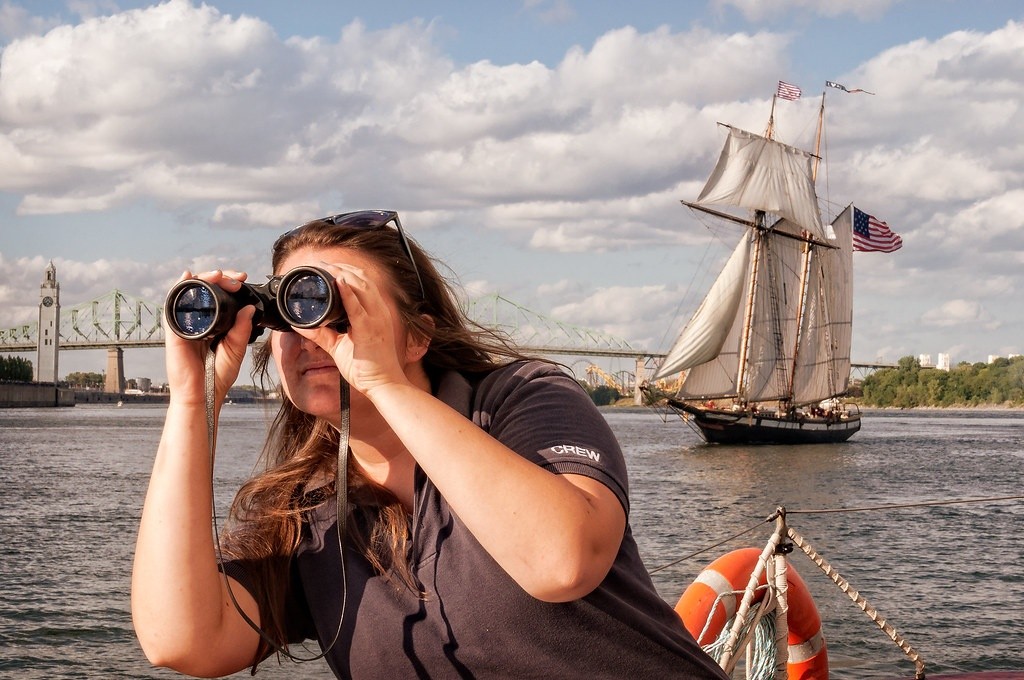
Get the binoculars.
[164,266,349,343]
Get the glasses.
[271,209,426,303]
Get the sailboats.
[639,91,863,446]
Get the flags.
[777,80,802,101]
[852,206,903,254]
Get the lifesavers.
[670,546,829,680]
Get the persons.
[751,402,757,413]
[131,209,732,680]
[830,405,849,419]
